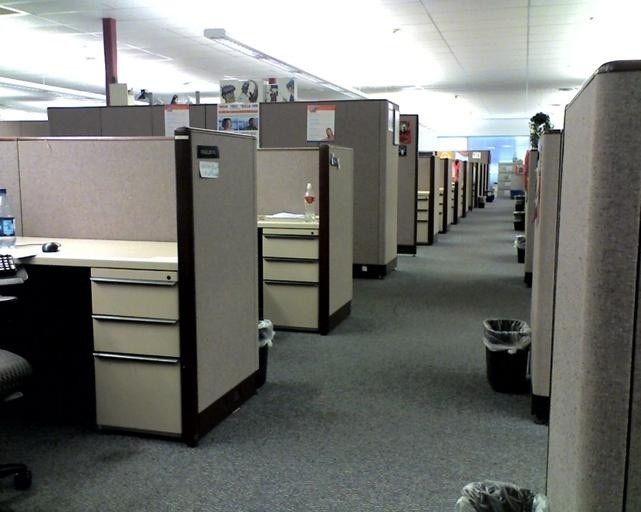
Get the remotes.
[42,242,57,252]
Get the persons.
[244,118,258,130]
[222,119,234,130]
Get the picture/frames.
[0,236,184,440]
[258,179,475,337]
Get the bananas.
[0,255,17,276]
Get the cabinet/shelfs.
[305,182,315,221]
[1,187,16,247]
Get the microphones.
[0,349,38,512]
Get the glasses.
[202,27,367,101]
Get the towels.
[514,195,525,211]
[486,190,494,202]
[483,318,532,394]
[478,196,486,208]
[512,211,525,231]
[455,479,549,512]
[257,319,273,386]
[516,235,526,263]
